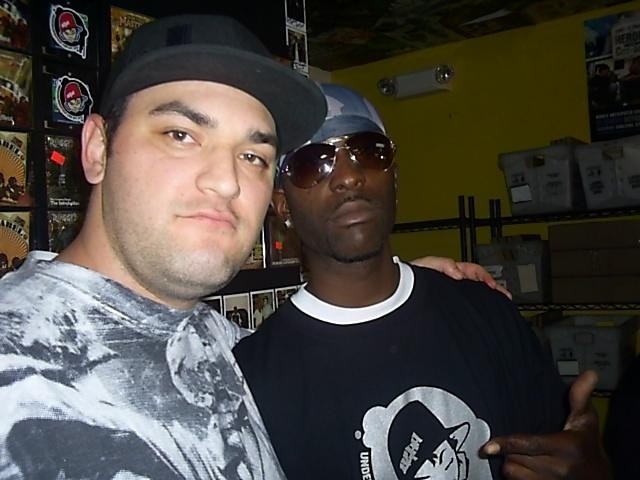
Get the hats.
[276,84,389,182]
[95,14,328,162]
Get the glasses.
[282,132,397,189]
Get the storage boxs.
[574,136,639,213]
[473,234,550,305]
[521,310,560,357]
[498,136,586,218]
[546,217,640,305]
[549,313,640,394]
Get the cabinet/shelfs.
[391,195,640,398]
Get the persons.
[233,86,615,480]
[0,15,512,480]
